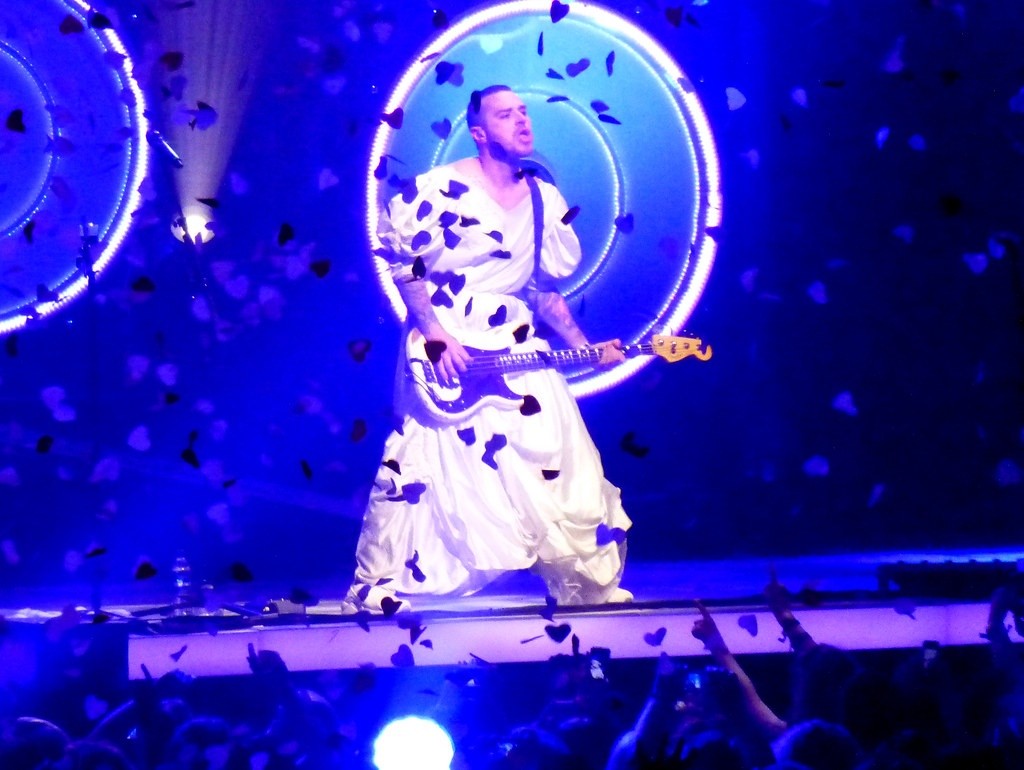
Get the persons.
[342,85,634,611]
[0,557,1022,770]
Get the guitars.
[404,321,713,426]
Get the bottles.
[172,556,190,616]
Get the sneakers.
[341,583,411,614]
[609,587,634,603]
